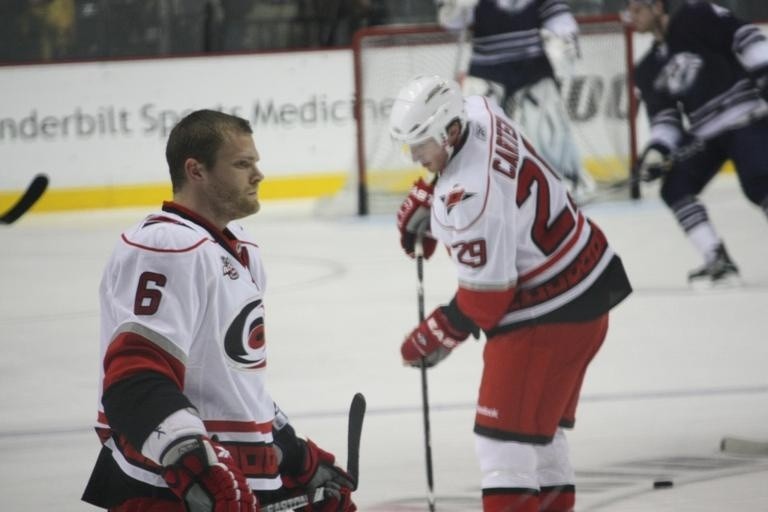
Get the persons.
[625,0,768,294]
[80,110,356,512]
[437,0,603,208]
[385,73,634,512]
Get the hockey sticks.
[257,392,366,511]
[0,174,49,225]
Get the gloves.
[636,142,673,181]
[402,305,465,367]
[397,177,436,258]
[279,439,343,501]
[162,435,259,512]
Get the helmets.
[389,75,467,146]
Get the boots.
[689,243,736,280]
